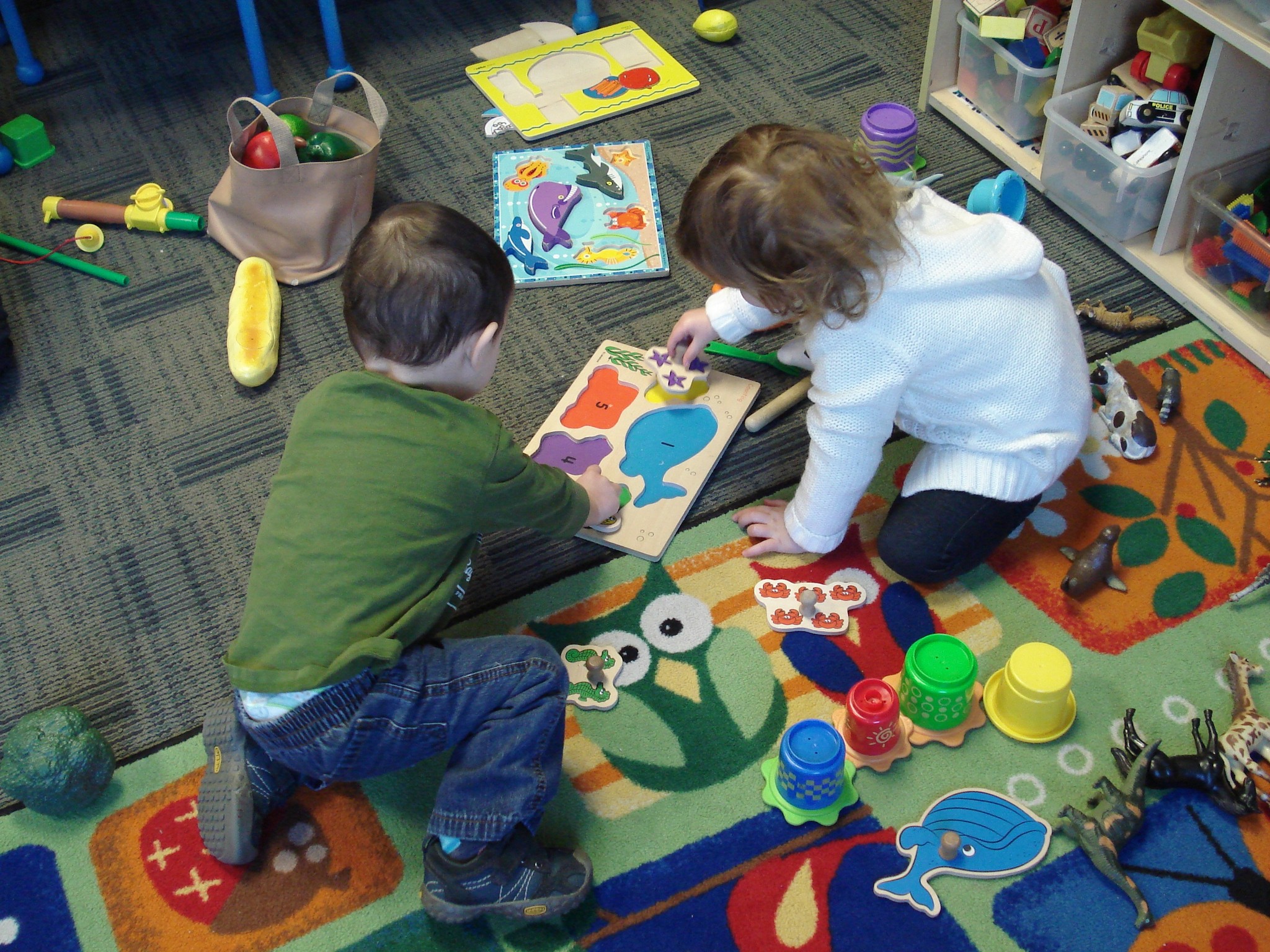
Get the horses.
[1112,707,1261,817]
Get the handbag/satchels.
[206,73,387,287]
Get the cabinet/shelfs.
[917,0,1269,381]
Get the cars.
[1120,89,1194,131]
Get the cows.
[1090,352,1157,461]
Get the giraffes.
[1214,651,1270,807]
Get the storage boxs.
[1191,146,1269,337]
[1037,79,1181,241]
[956,8,1059,141]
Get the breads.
[225,256,282,387]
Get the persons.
[664,121,1092,587]
[196,201,622,926]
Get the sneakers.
[198,698,274,863]
[421,829,593,923]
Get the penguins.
[1059,524,1128,597]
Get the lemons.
[693,9,738,42]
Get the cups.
[774,719,845,810]
[966,170,1028,224]
[983,641,1076,744]
[897,633,977,731]
[841,678,901,755]
[857,102,918,172]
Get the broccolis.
[0,707,115,817]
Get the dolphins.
[501,217,549,275]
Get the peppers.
[243,114,363,168]
